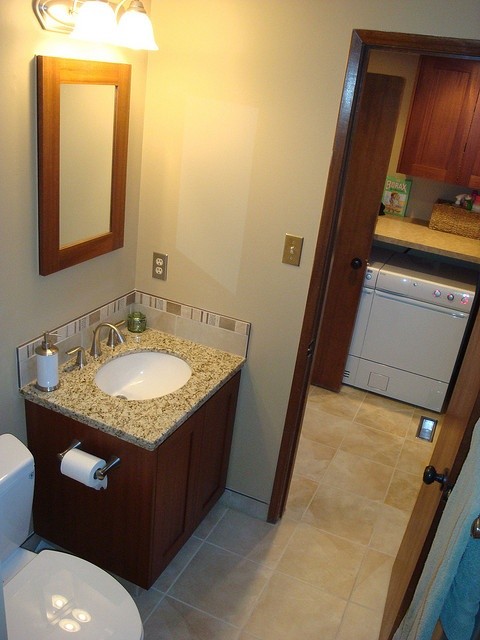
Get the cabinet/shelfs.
[374,216,480,263]
[25,369,242,591]
[395,52,479,189]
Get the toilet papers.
[60,448,109,491]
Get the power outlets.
[151,250,169,282]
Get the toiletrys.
[34,331,63,392]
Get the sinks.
[94,348,193,401]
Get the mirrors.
[35,54,132,277]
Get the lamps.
[32,0,160,56]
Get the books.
[381,176,412,216]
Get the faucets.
[89,322,127,357]
